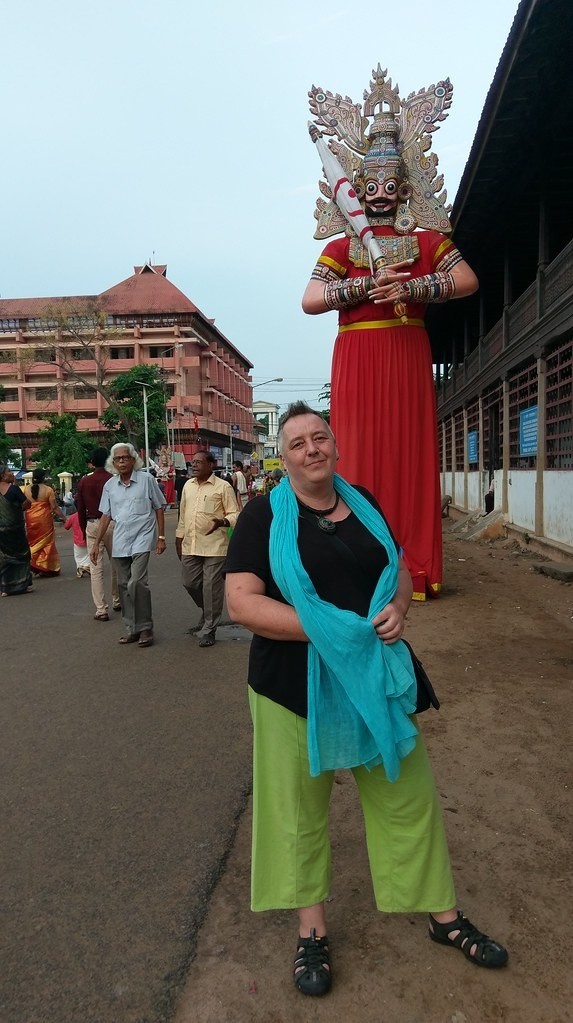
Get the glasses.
[113,455,132,463]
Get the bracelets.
[159,536,165,539]
[223,519,226,525]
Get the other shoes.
[75,570,84,578]
[188,625,203,635]
[25,585,33,592]
[199,631,216,647]
[1,592,8,597]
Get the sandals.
[426,909,510,971]
[294,926,335,995]
[113,605,121,611]
[138,629,154,647]
[118,632,138,644]
[94,613,110,621]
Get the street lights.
[251,377,283,466]
[161,344,184,509]
[135,372,154,473]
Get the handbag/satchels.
[400,642,441,714]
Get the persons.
[53,488,65,507]
[174,450,238,647]
[63,489,77,512]
[302,62,479,601]
[220,399,508,996]
[243,465,255,482]
[174,469,189,521]
[24,469,67,577]
[90,443,167,646]
[232,460,249,507]
[0,465,33,597]
[157,478,166,493]
[76,447,121,621]
[223,477,244,508]
[64,499,91,578]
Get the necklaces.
[296,489,340,531]
